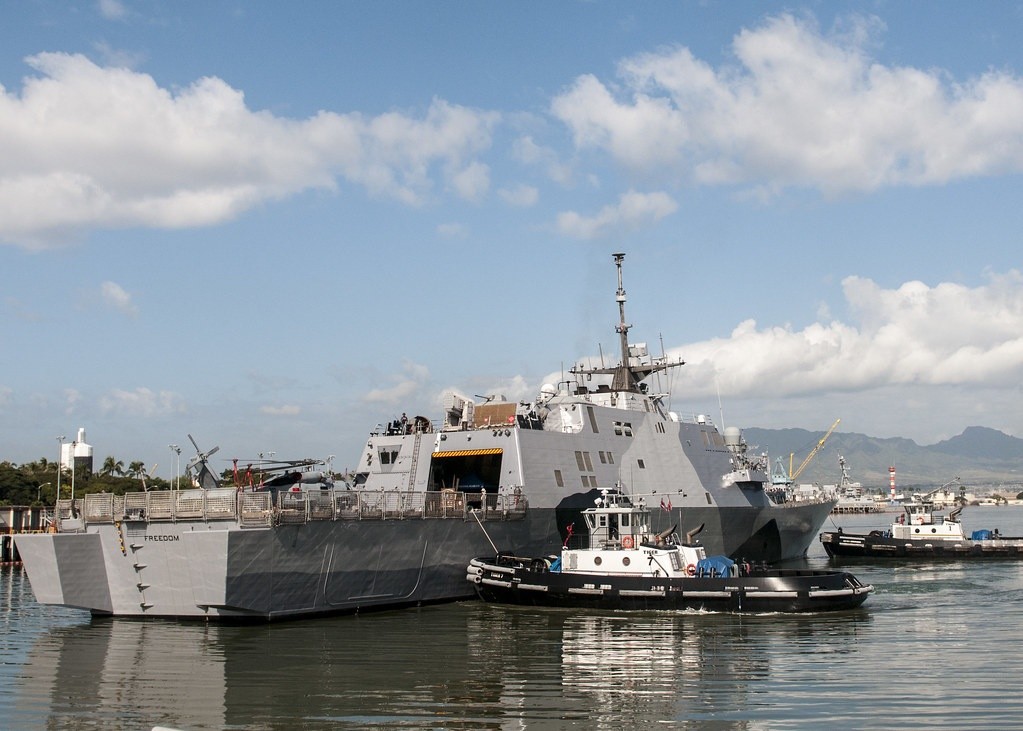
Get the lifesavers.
[623,537,633,548]
[917,517,924,524]
[687,564,696,575]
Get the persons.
[415,415,430,433]
[400,413,408,425]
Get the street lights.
[56,436,65,523]
[257,452,264,488]
[38,482,52,506]
[175,448,181,512]
[168,444,178,522]
[266,451,276,476]
[71,440,75,519]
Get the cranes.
[771,419,841,485]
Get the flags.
[660,500,668,512]
[668,498,672,511]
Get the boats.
[13,247,840,624]
[819,475,1023,560]
[465,467,876,615]
